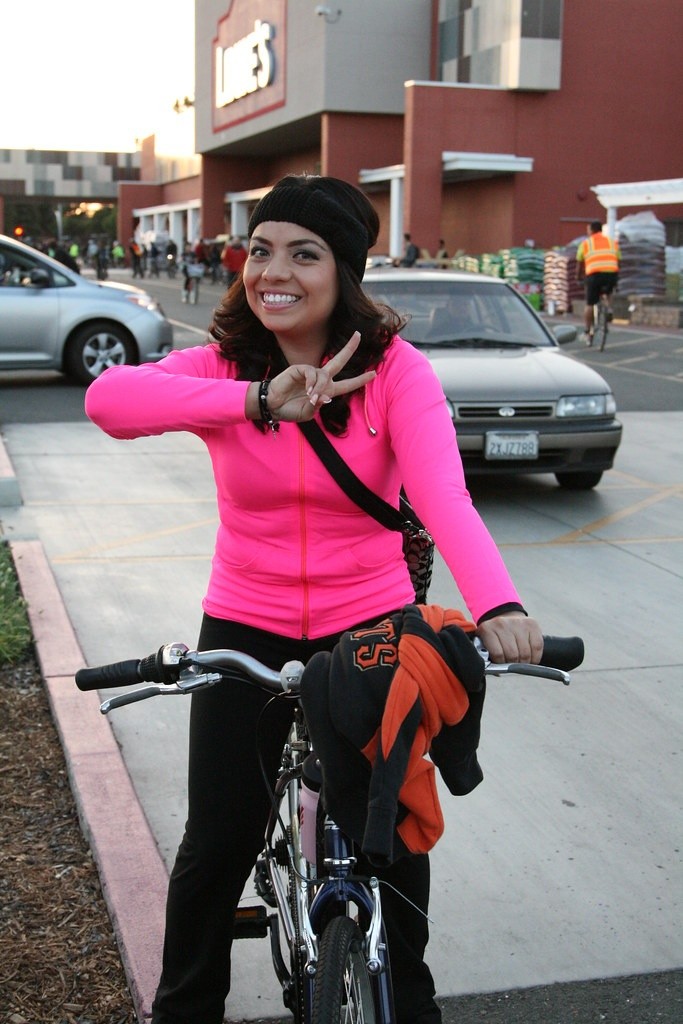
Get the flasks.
[301,752,323,867]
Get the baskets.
[187,264,204,279]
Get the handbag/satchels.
[396,491,435,604]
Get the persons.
[574,220,622,343]
[0,227,453,306]
[80,170,547,1024]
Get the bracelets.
[258,378,281,441]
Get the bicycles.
[71,608,585,1023]
[183,265,204,306]
[576,285,616,349]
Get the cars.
[0,233,174,388]
[356,267,624,495]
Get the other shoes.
[606,309,613,323]
[395,994,441,1024]
[580,333,592,346]
